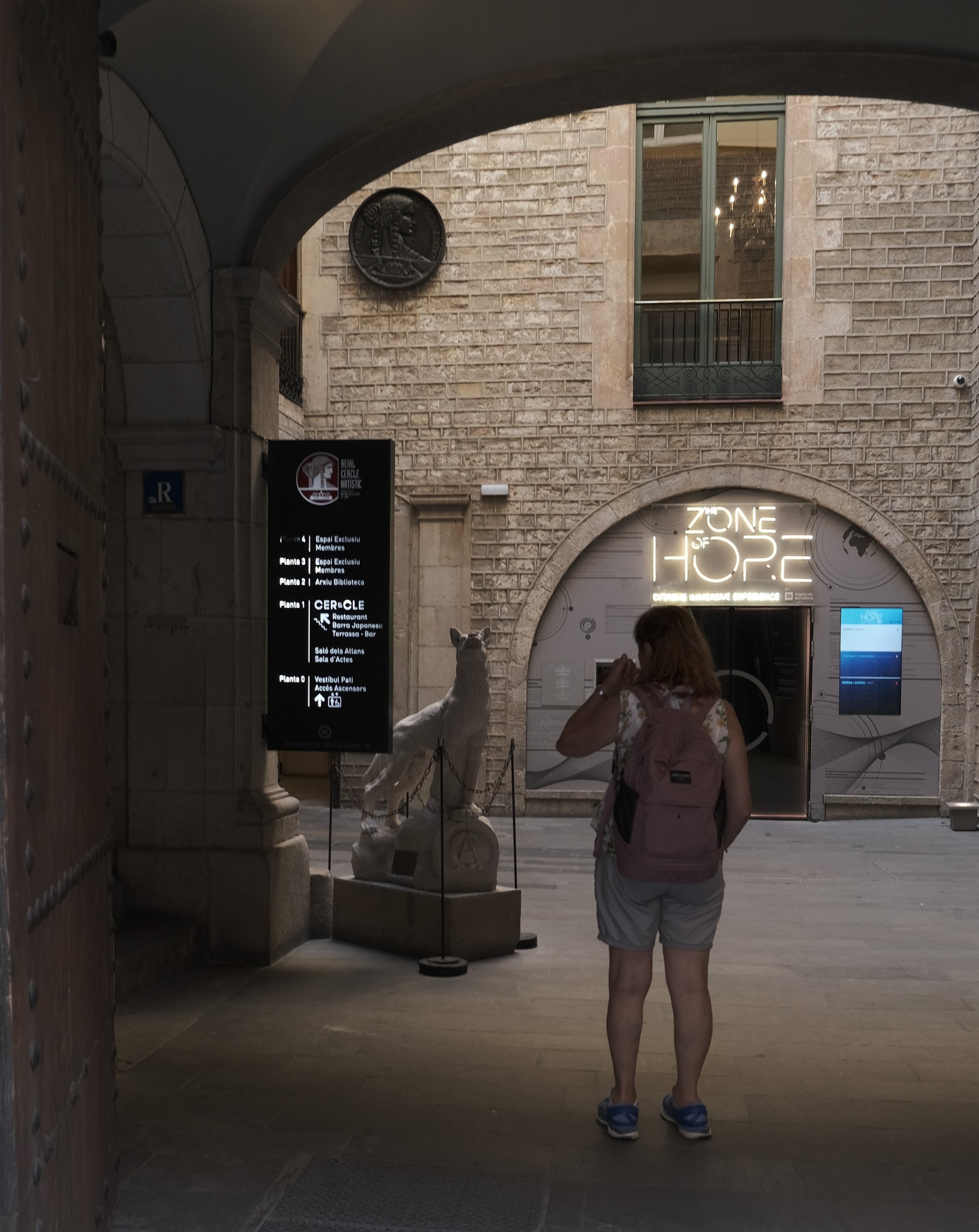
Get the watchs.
[594,685,609,701]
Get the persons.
[555,604,751,1140]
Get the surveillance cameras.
[953,374,966,388]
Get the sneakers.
[596,1086,639,1140]
[661,1085,712,1140]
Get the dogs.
[357,626,491,834]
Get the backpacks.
[611,683,727,883]
